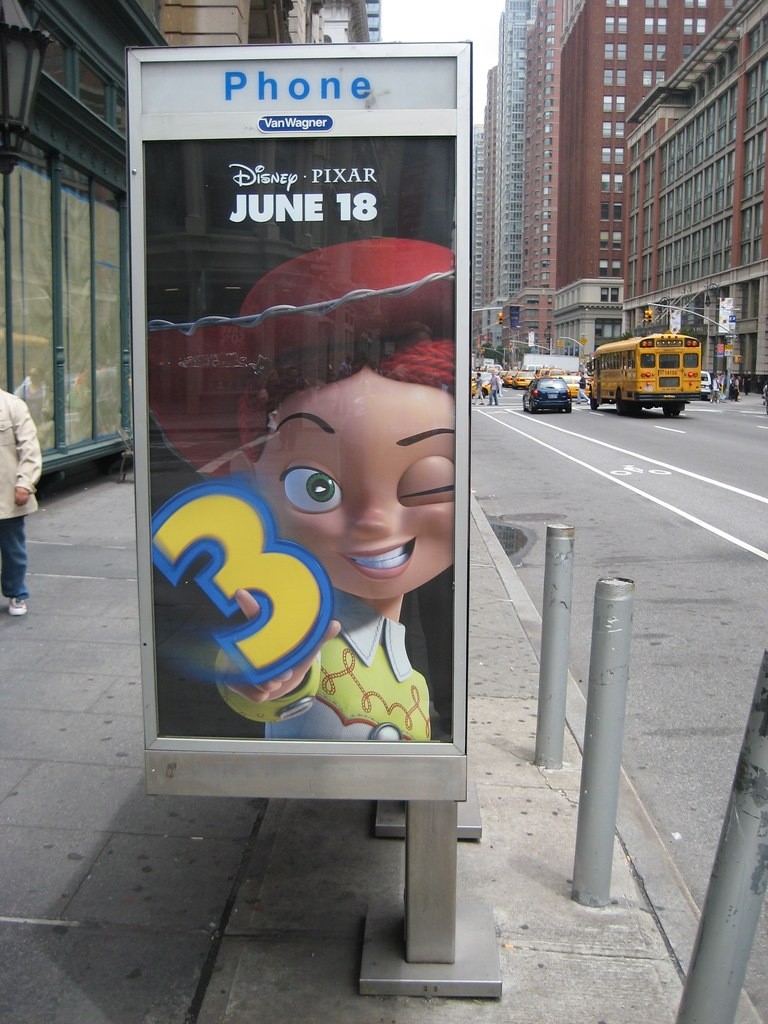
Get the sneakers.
[8,597,27,615]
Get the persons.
[710,371,752,404]
[472,371,503,405]
[14,355,120,447]
[575,372,590,404]
[0,390,45,615]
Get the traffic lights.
[645,310,650,324]
[557,339,565,350]
[497,313,504,326]
[647,310,653,324]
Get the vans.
[701,370,712,399]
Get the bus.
[585,333,705,416]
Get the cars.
[493,364,591,399]
[471,379,490,399]
[521,378,573,414]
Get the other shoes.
[575,402,581,405]
[472,401,475,404]
[479,403,484,405]
